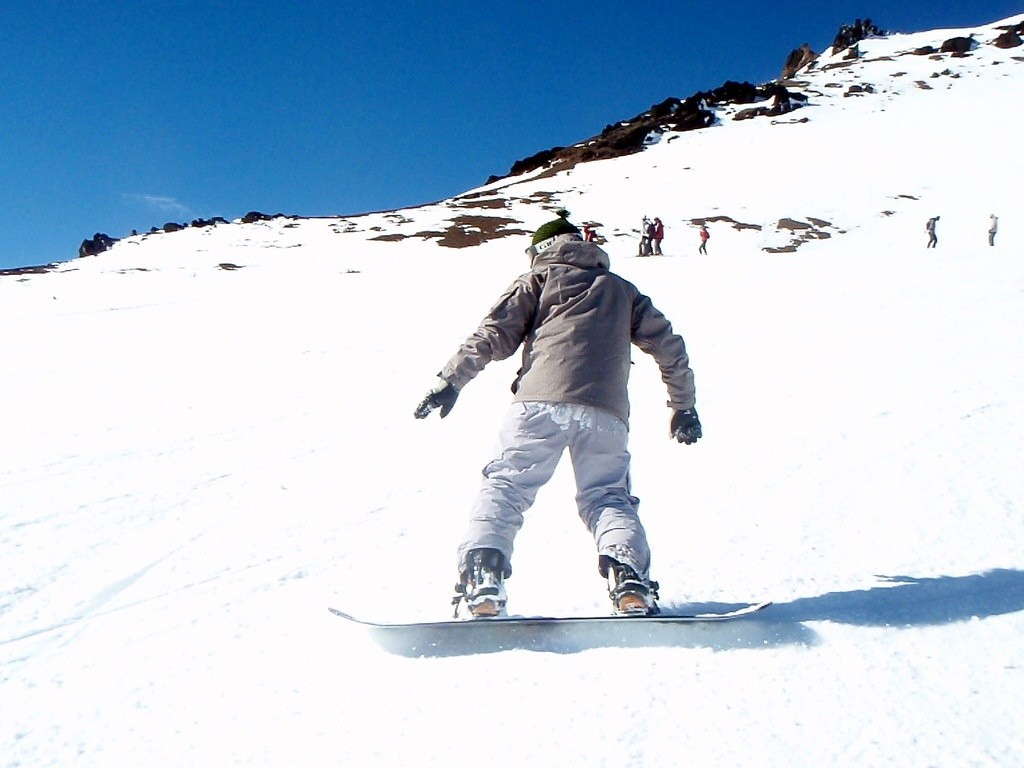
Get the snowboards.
[325,600,777,629]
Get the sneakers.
[607,564,661,615]
[460,557,509,617]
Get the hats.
[532,218,583,254]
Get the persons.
[699,224,710,255]
[413,210,704,618]
[926,216,940,248]
[988,213,998,246]
[636,216,664,257]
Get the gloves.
[413,384,456,420]
[671,407,702,446]
